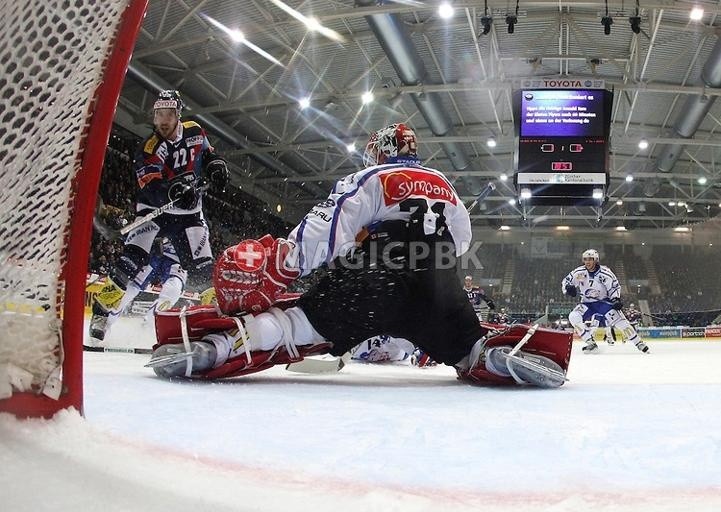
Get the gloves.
[212,233,302,317]
[565,284,576,297]
[164,177,200,211]
[486,300,495,310]
[202,153,232,196]
[610,297,623,311]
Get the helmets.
[365,122,419,164]
[152,88,183,114]
[582,247,600,263]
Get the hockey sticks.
[92,180,210,241]
[287,341,361,374]
[577,292,672,322]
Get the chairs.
[461,236,721,330]
[87,124,298,309]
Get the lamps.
[480,1,645,38]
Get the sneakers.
[141,310,158,340]
[636,340,649,353]
[88,297,112,341]
[606,336,614,345]
[582,337,598,351]
[483,345,566,389]
[151,341,216,382]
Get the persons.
[149,120,565,391]
[461,248,720,354]
[87,88,326,341]
[346,332,438,369]
